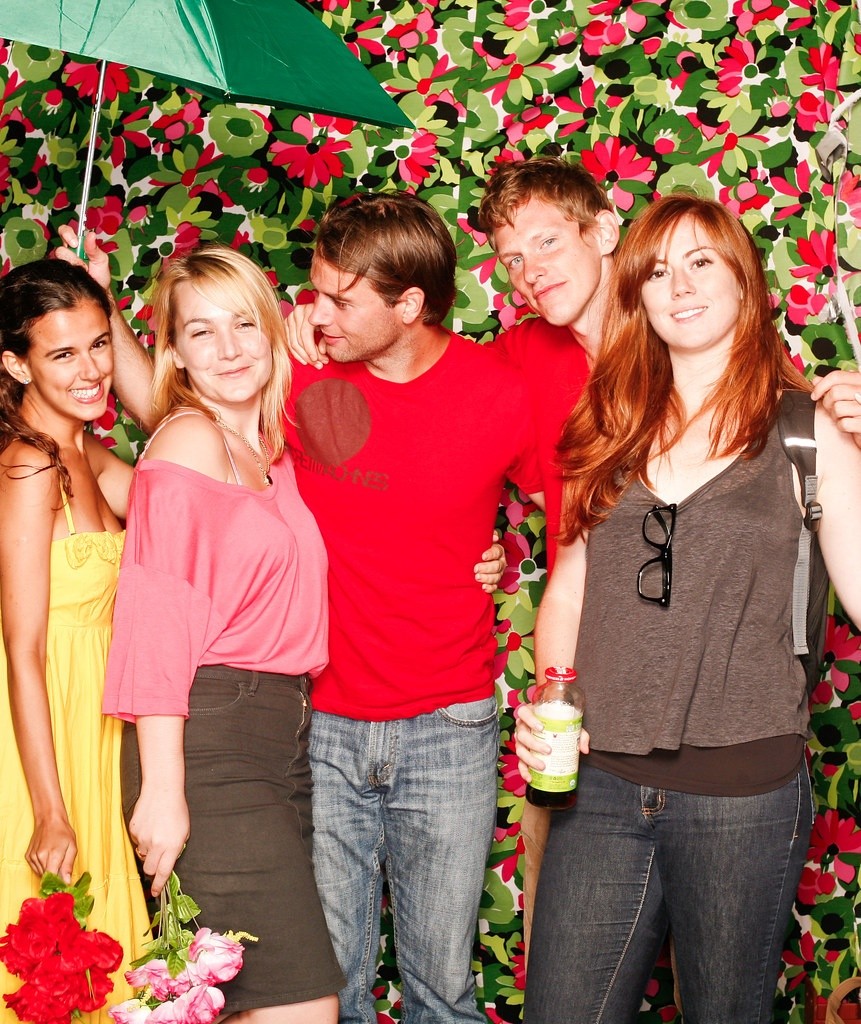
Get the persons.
[513,196,861,1024]
[285,157,640,971]
[52,190,545,1024]
[0,259,154,1024]
[100,246,506,1024]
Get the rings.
[137,847,147,857]
[855,392,861,405]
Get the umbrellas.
[0,0,415,256]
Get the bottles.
[526,667,585,810]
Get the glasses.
[637,504,678,607]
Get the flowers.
[0,871,261,1024]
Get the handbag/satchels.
[780,389,829,696]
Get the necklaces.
[217,419,274,486]
